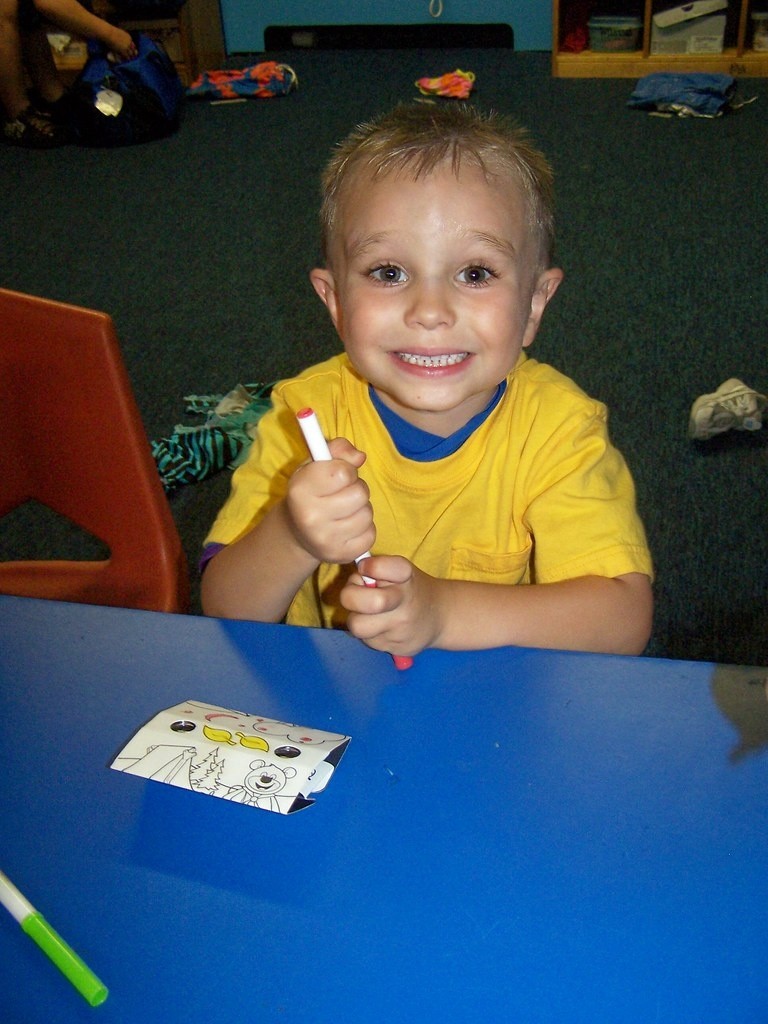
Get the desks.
[0,595,768,1024]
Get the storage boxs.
[588,17,638,54]
[650,0,728,55]
[752,13,768,52]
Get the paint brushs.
[299,407,414,669]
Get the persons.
[197,104,654,655]
[0,0,138,145]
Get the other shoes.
[42,86,78,115]
[3,106,62,148]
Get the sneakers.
[689,379,767,440]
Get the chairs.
[0,290,187,613]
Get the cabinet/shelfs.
[551,0,768,78]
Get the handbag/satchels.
[79,30,183,137]
[626,72,761,119]
[186,61,298,99]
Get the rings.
[130,42,135,50]
